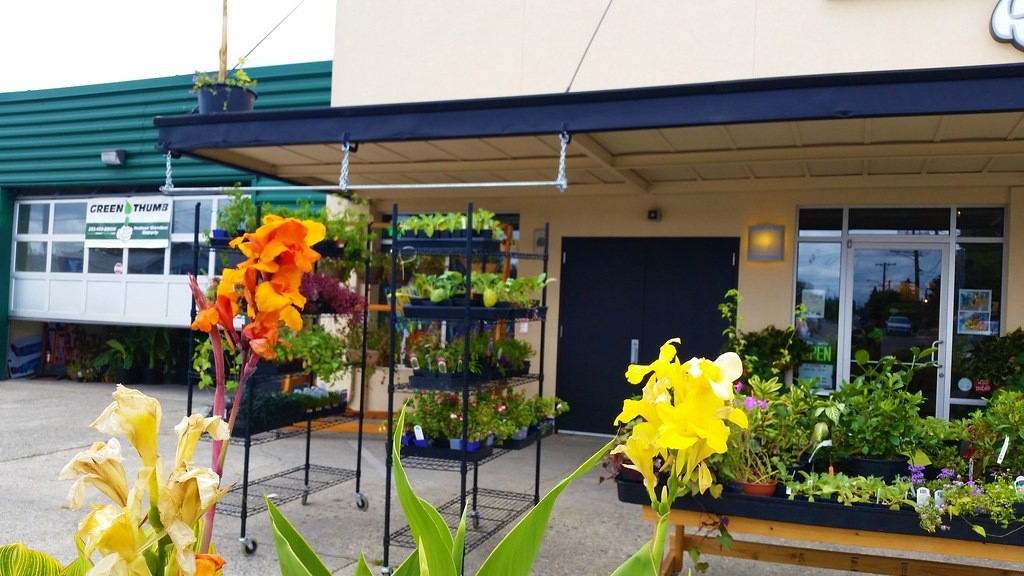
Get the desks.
[640,505,1024,576]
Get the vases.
[195,82,257,113]
[741,480,775,496]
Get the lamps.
[99,149,125,167]
[746,224,784,260]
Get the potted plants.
[66,327,180,383]
[596,288,1023,546]
[190,181,569,452]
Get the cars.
[885,316,914,336]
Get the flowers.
[187,57,256,110]
[899,465,986,539]
[963,423,1003,462]
[731,382,780,485]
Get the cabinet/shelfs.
[380,202,549,576]
[191,200,376,556]
